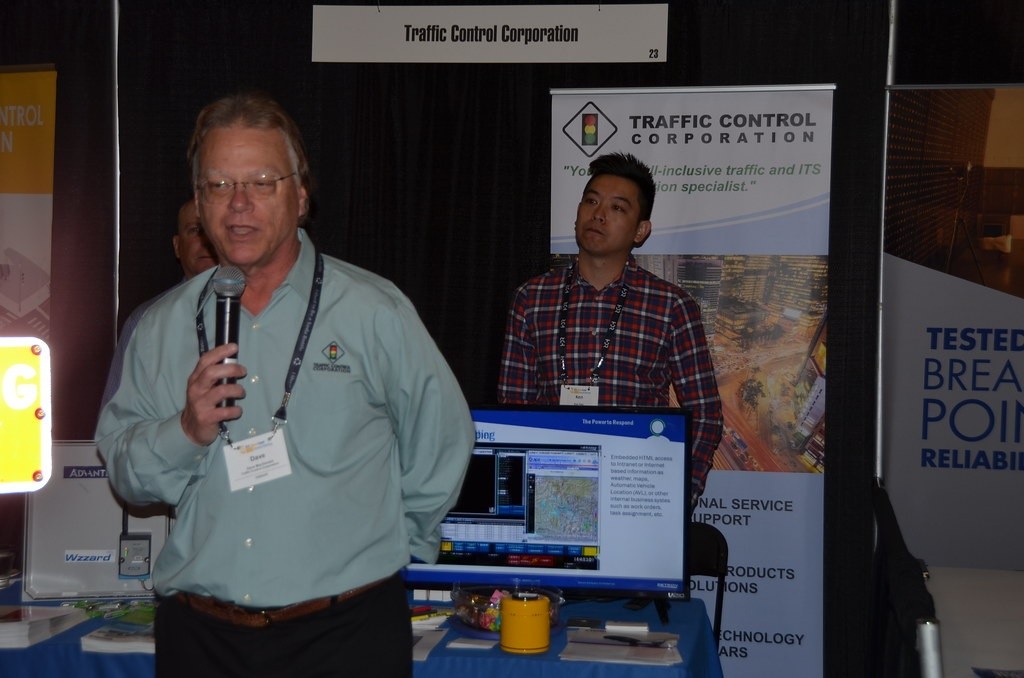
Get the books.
[0,605,80,649]
[81,621,155,654]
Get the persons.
[98,192,220,420]
[495,151,723,516]
[94,92,477,678]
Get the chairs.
[688,521,728,649]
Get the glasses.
[198,173,296,206]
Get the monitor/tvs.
[404,404,694,602]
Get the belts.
[175,571,395,629]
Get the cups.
[500,593,550,654]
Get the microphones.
[211,264,245,419]
